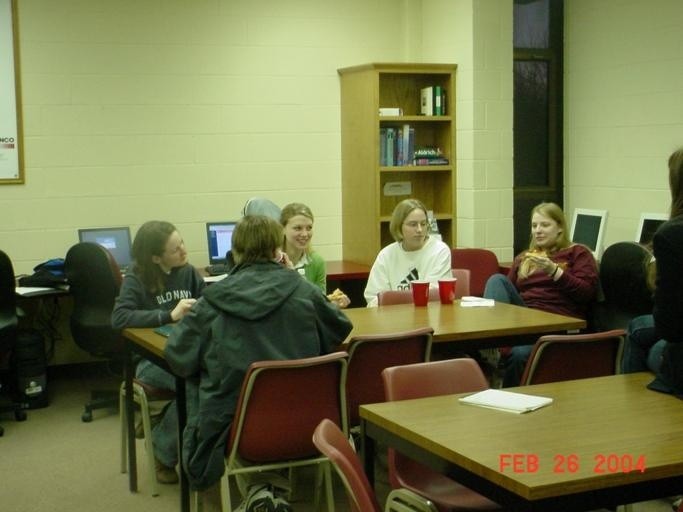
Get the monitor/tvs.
[634,212,671,246]
[206,220,236,264]
[78,227,136,266]
[572,207,609,259]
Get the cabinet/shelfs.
[337,62,457,264]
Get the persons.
[484,202,603,389]
[620,148,683,399]
[112,221,209,484]
[163,214,354,491]
[279,203,351,308]
[363,199,453,308]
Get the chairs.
[451,248,498,298]
[312,419,379,512]
[119,337,176,497]
[380,357,502,511]
[344,329,434,429]
[520,329,628,386]
[189,350,356,512]
[64,243,121,424]
[0,251,27,434]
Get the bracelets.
[550,265,559,278]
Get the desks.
[123,297,588,512]
[359,371,682,512]
[196,258,371,289]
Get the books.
[442,90,446,115]
[380,124,415,166]
[414,158,450,165]
[420,86,433,116]
[434,86,441,116]
[379,107,403,116]
[458,389,554,414]
[414,145,440,157]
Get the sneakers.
[232,484,275,512]
[144,440,179,485]
[135,412,161,440]
[274,498,294,512]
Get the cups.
[409,281,430,307]
[439,278,459,303]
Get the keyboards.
[203,267,234,275]
